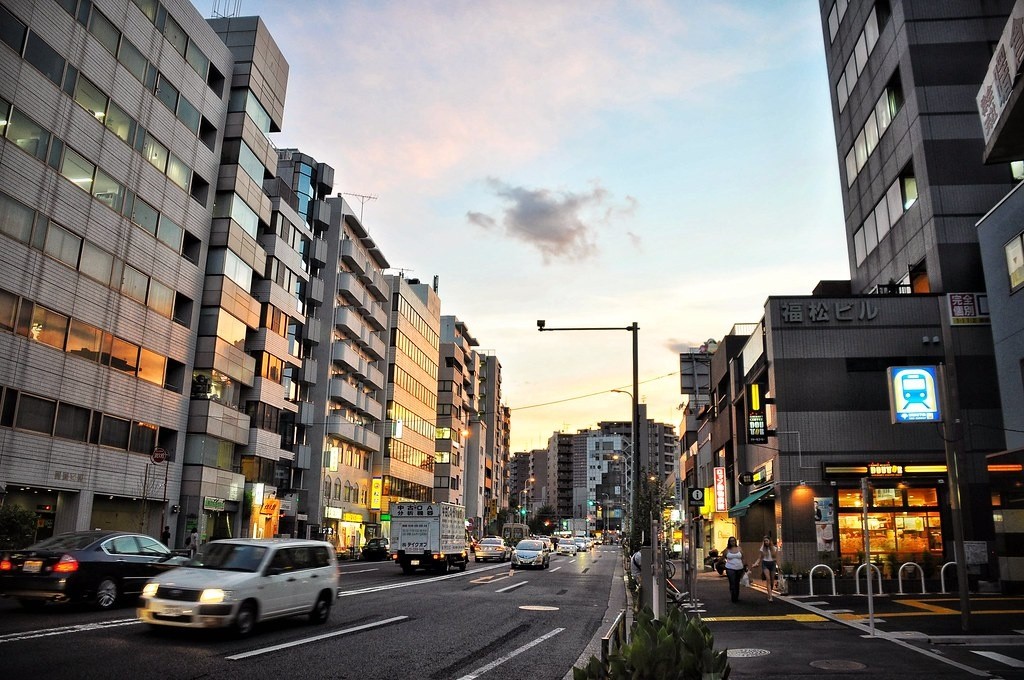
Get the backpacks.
[192,541,195,546]
[185,537,191,545]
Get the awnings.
[728,486,776,518]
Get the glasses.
[169,528,170,529]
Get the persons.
[159,526,171,547]
[752,536,777,603]
[814,501,834,522]
[630,541,689,603]
[189,527,199,549]
[719,536,748,603]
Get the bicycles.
[664,560,676,579]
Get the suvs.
[136,536,341,642]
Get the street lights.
[612,388,635,535]
[518,489,528,524]
[602,493,610,536]
[612,454,628,514]
[524,477,535,525]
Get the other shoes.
[767,596,773,602]
[732,598,738,604]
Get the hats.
[194,533,198,537]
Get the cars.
[574,536,588,552]
[362,537,390,561]
[532,532,604,551]
[0,530,193,614]
[474,534,513,563]
[555,537,578,556]
[538,538,555,553]
[510,540,551,570]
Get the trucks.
[389,500,469,575]
[502,523,534,547]
